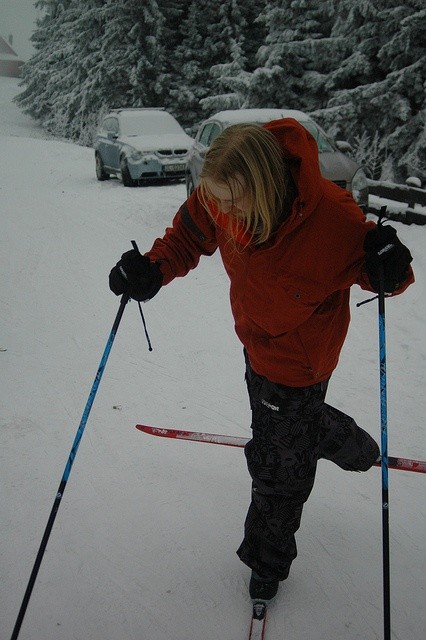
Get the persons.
[109,117,412,605]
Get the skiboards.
[248,602,267,640]
[135,424,425,474]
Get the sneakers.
[248,567,280,600]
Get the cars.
[94,107,193,186]
[184,108,368,213]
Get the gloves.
[109,249,163,303]
[360,223,414,293]
